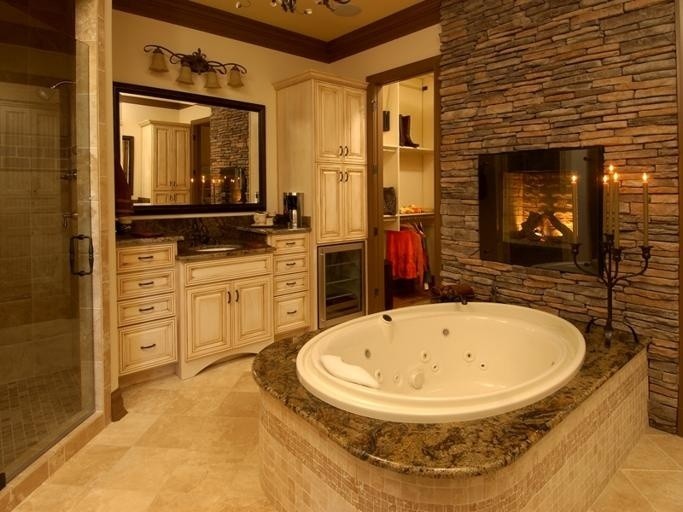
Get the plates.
[250,224,275,228]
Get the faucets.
[454,294,468,305]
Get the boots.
[399,113,419,148]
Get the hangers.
[398,217,428,239]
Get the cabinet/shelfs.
[242,231,310,344]
[114,241,178,376]
[271,68,369,244]
[382,74,436,291]
[315,239,368,329]
[176,253,275,380]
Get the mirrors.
[114,81,268,216]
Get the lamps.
[143,42,249,88]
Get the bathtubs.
[295,301,587,425]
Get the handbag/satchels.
[382,187,397,216]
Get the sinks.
[189,245,240,252]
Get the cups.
[252,214,273,225]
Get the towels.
[320,354,379,389]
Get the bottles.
[287,191,304,229]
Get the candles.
[200,175,217,202]
[568,163,649,249]
